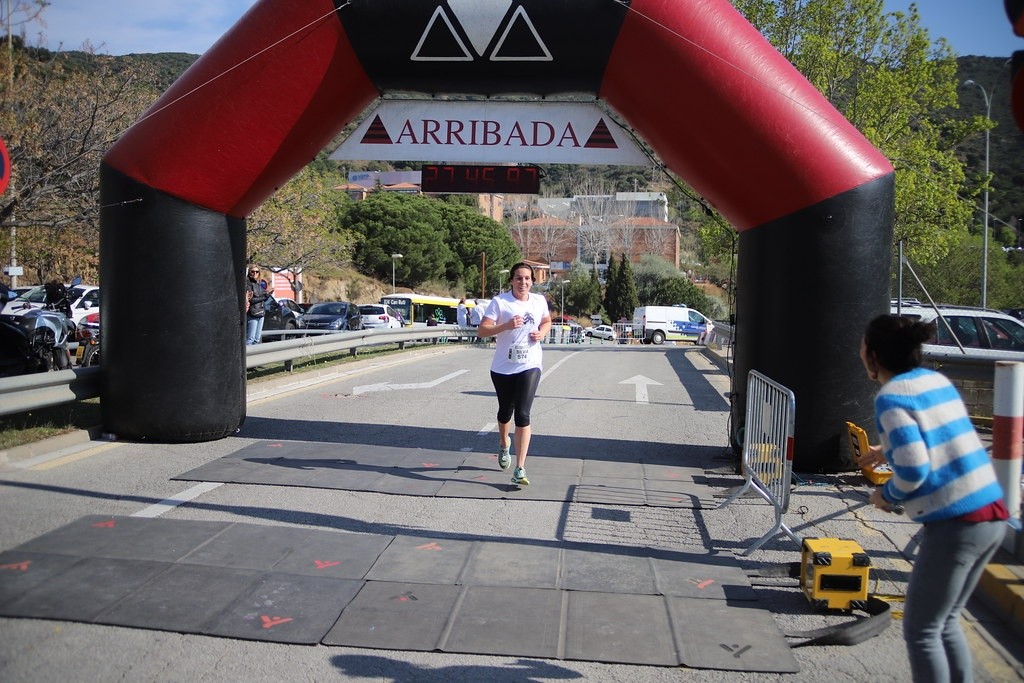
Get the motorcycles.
[77,312,99,367]
[11,277,81,371]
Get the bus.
[378,293,476,327]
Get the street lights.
[562,280,571,326]
[392,253,404,294]
[961,57,1012,310]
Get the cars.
[359,304,406,329]
[584,325,617,341]
[1,284,99,329]
[299,302,363,338]
[274,297,305,328]
[553,317,575,325]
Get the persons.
[457,298,485,342]
[427,315,438,326]
[246,265,274,346]
[477,262,551,485]
[0,264,19,297]
[44,268,82,318]
[859,314,1010,683]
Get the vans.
[633,304,717,345]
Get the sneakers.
[498,436,512,470]
[511,466,530,485]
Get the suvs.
[886,299,1024,377]
[261,294,298,343]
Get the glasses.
[251,270,260,273]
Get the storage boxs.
[745,443,783,484]
[798,537,871,611]
[848,421,893,484]
